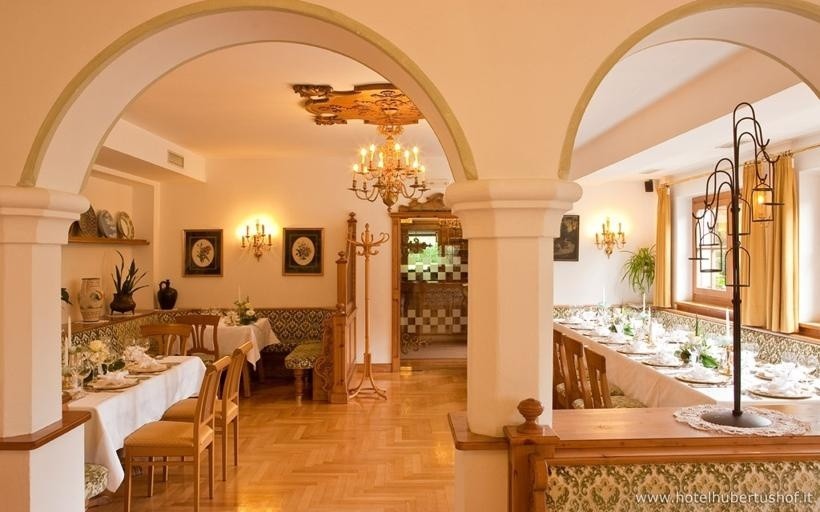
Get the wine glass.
[68,336,151,391]
[200,303,220,316]
[568,301,817,391]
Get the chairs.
[175,315,220,360]
[124,356,232,512]
[553,329,647,409]
[162,341,253,481]
[140,323,192,356]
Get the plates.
[292,237,315,265]
[559,318,814,399]
[191,239,214,268]
[78,205,135,240]
[93,363,172,389]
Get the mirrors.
[406,231,440,266]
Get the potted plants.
[110,249,149,315]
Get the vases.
[78,278,105,322]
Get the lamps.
[595,221,626,259]
[241,224,272,262]
[349,115,430,212]
[690,102,784,428]
[402,238,432,254]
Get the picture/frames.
[180,229,223,277]
[282,228,325,276]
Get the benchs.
[61,309,335,512]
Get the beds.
[62,355,219,494]
